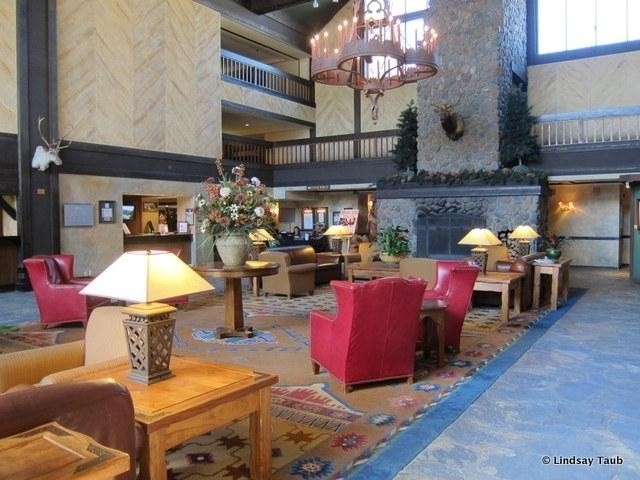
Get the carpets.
[1,277,594,479]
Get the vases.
[212,231,253,267]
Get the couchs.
[266,245,343,285]
[494,251,547,311]
[0,377,136,479]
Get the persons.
[159,215,169,233]
[292,227,301,239]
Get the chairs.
[416,260,479,352]
[22,253,111,331]
[257,251,317,300]
[343,242,380,281]
[159,249,189,311]
[308,275,426,394]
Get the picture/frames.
[62,202,96,227]
[99,200,116,224]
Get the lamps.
[306,0,442,128]
[322,225,352,254]
[77,248,221,386]
[247,228,275,260]
[456,227,503,274]
[508,224,542,257]
[557,200,577,214]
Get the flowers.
[190,158,279,254]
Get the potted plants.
[543,232,566,264]
[368,225,412,265]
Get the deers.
[31,114,73,171]
[426,94,466,142]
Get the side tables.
[421,301,446,369]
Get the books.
[533,258,559,264]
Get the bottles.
[159,220,168,235]
[305,233,309,241]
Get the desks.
[532,257,573,312]
[1,416,129,479]
[36,355,278,479]
[472,271,527,322]
[191,261,281,339]
